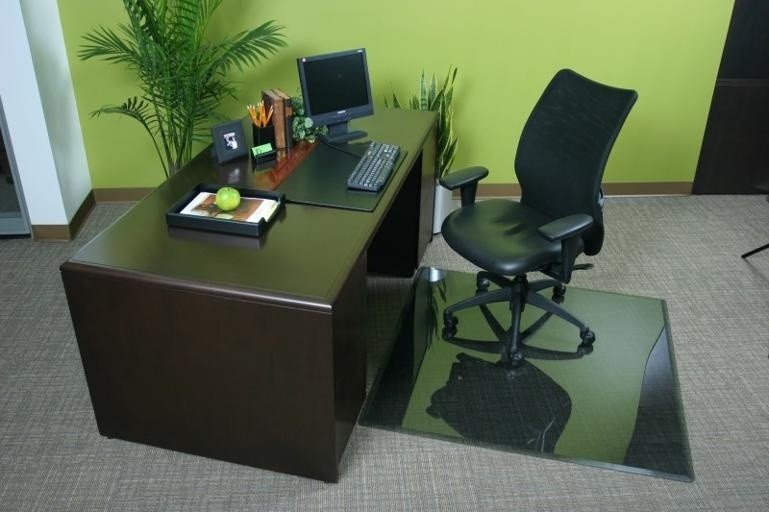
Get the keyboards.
[345,141,400,193]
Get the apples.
[215,186,240,210]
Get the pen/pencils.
[246,100,273,128]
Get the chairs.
[439,68,638,369]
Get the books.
[261,88,294,148]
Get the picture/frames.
[209,119,249,166]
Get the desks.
[59,108,443,485]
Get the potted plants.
[382,64,460,236]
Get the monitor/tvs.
[296,46,374,145]
